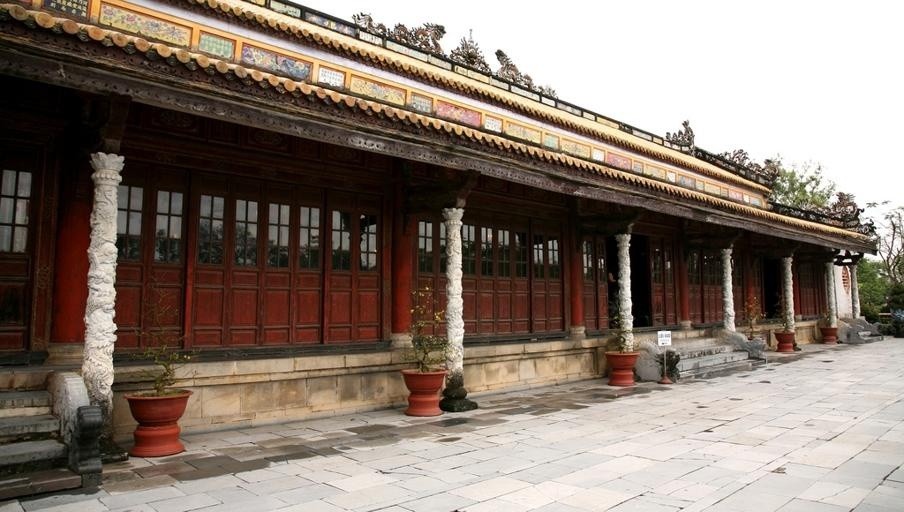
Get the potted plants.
[400,277,446,417]
[773,310,795,351]
[122,273,194,457]
[603,292,642,386]
[820,309,838,344]
